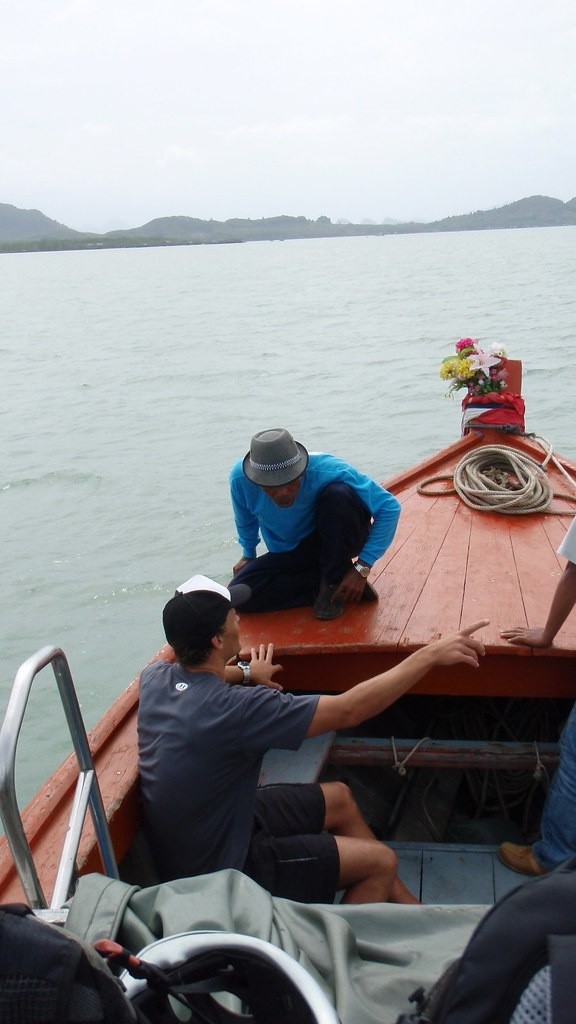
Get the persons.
[228,428,402,618]
[499,516,575,877]
[137,591,490,903]
[0,904,135,1024]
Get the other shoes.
[497,841,551,877]
[360,581,378,601]
[312,586,345,619]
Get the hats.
[162,574,251,645]
[242,428,309,488]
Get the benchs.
[258,730,337,787]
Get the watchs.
[238,661,251,684]
[353,561,371,578]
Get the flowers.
[440,338,509,400]
